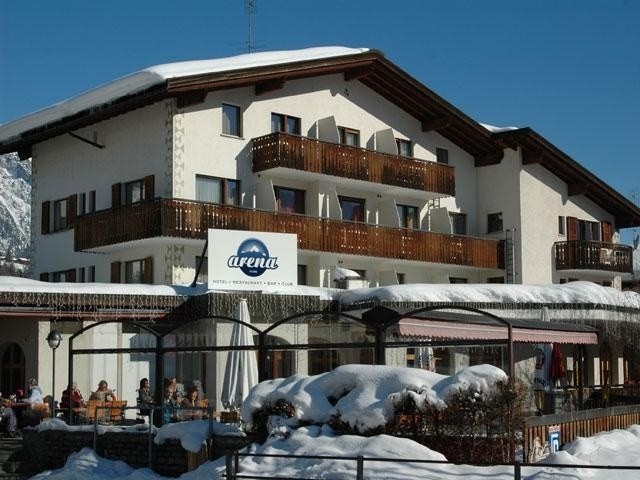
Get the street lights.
[44,330,65,421]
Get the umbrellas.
[549,343,567,388]
[221,297,260,412]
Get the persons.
[138,378,160,429]
[59,381,84,415]
[92,380,116,401]
[17,377,44,415]
[163,376,208,424]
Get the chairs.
[1,389,241,431]
[566,378,640,412]
